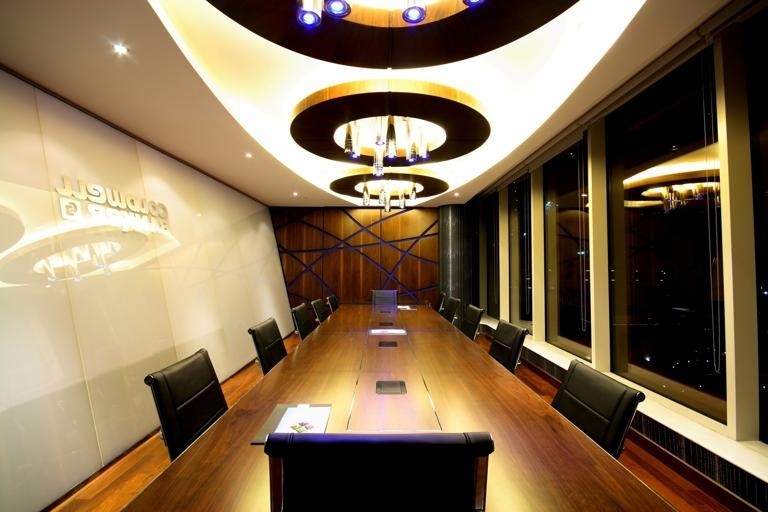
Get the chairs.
[488,319,530,374]
[292,294,339,341]
[433,290,485,342]
[371,290,398,305]
[144,347,229,464]
[549,359,646,459]
[263,430,495,512]
[248,316,288,376]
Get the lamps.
[288,76,492,177]
[204,0,582,71]
[329,166,452,214]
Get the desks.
[122,305,678,511]
